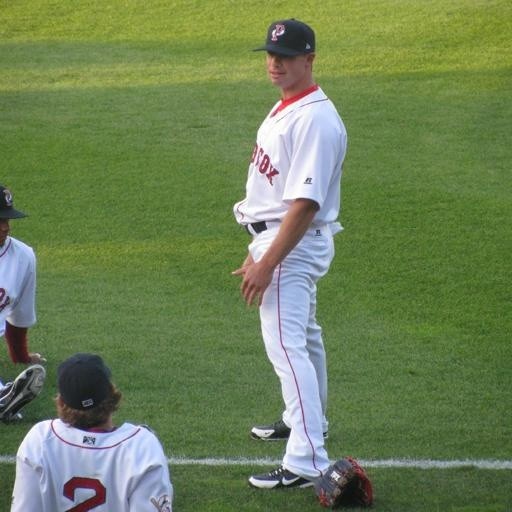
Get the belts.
[242,222,267,236]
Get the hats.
[0,186,28,219]
[252,17,315,57]
[57,353,111,409]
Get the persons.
[232,20,348,491]
[11,353,173,512]
[0,186,47,425]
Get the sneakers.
[0,365,46,423]
[249,419,329,441]
[247,466,315,491]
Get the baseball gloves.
[317,458,373,509]
